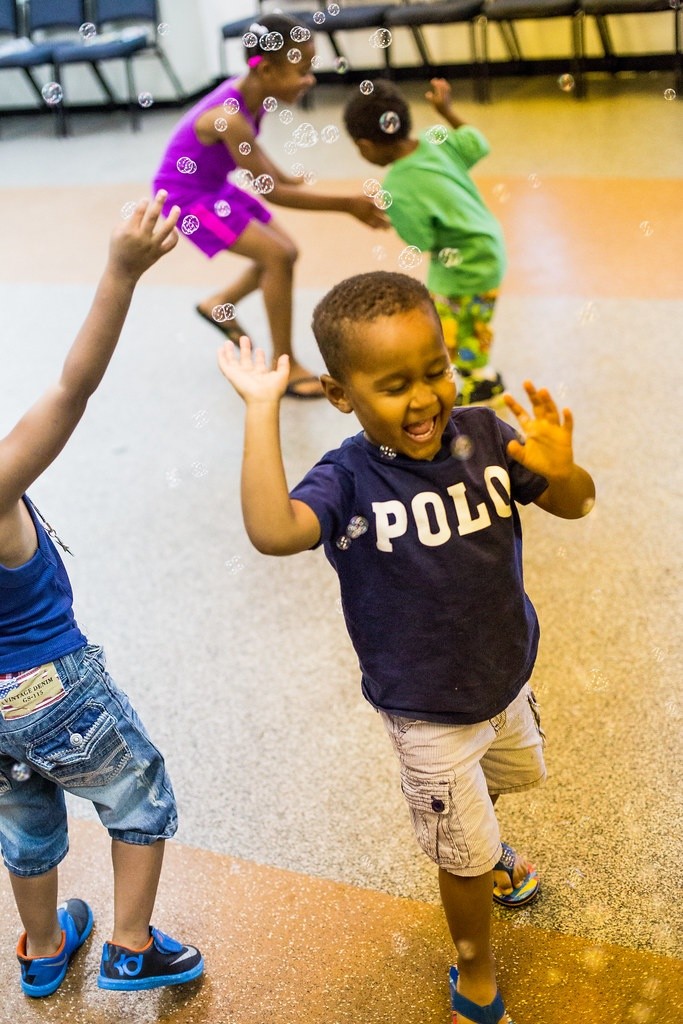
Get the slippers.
[284,376,327,398]
[449,963,514,1024]
[196,303,253,351]
[492,842,539,906]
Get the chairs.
[0,0,683,138]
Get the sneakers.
[453,366,508,407]
[17,898,93,997]
[98,926,204,991]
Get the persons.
[344,79,505,409]
[1,188,203,997]
[217,270,596,1024]
[153,13,392,399]
